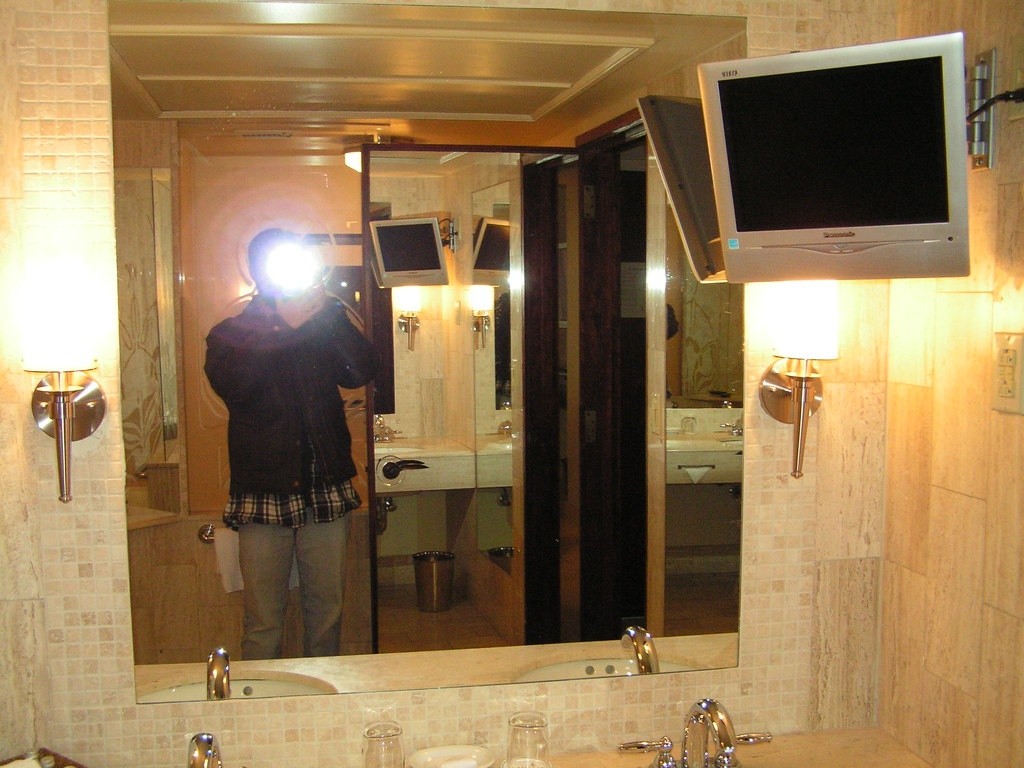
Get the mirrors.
[469,179,514,578]
[664,194,743,408]
[105,0,745,707]
[115,162,181,521]
[356,142,582,655]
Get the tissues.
[676,462,717,483]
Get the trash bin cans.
[413,552,454,613]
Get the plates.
[409,744,495,768]
[500,757,552,768]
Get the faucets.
[204,645,232,700]
[720,422,743,436]
[620,624,659,673]
[680,697,741,768]
[374,414,402,443]
[188,731,222,767]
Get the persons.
[203,228,376,660]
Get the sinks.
[511,648,708,687]
[721,439,743,445]
[374,446,423,455]
[137,667,337,704]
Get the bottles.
[362,720,406,768]
[508,712,549,768]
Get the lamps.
[470,285,494,349]
[399,286,421,350]
[23,358,105,503]
[760,278,841,480]
[341,151,364,174]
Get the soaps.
[441,757,477,768]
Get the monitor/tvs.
[696,30,971,284]
[636,94,729,284]
[368,218,450,289]
[471,217,510,284]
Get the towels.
[213,526,301,595]
